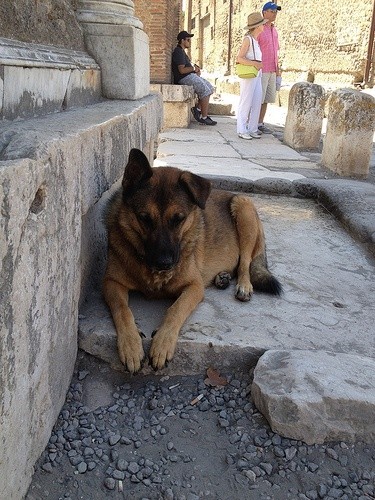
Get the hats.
[244,11,269,29]
[176,31,194,41]
[261,1,282,10]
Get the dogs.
[102,148,283,376]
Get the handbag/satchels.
[235,59,262,79]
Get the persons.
[172,30,217,126]
[236,13,261,140]
[259,2,282,129]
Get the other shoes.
[250,133,262,139]
[239,133,253,140]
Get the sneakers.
[257,130,263,135]
[191,106,202,123]
[258,125,274,134]
[200,116,218,125]
